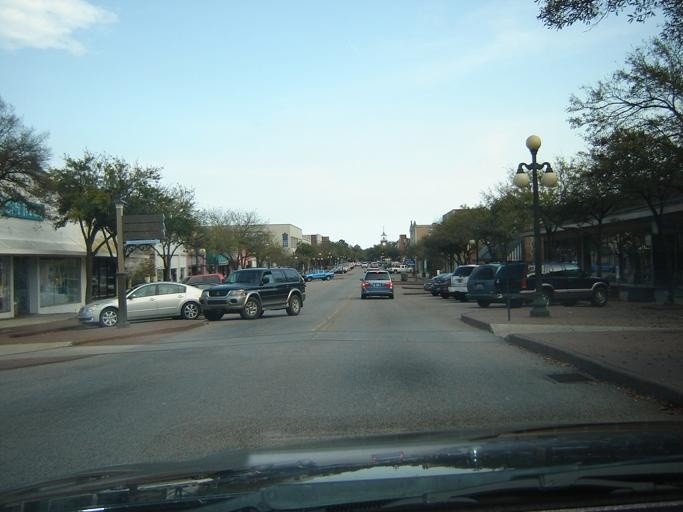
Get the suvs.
[494,262,615,307]
[446,263,481,300]
[359,271,395,300]
[199,265,307,324]
[465,260,527,307]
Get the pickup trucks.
[301,269,334,283]
[387,264,413,274]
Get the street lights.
[289,247,339,270]
[512,136,561,317]
[112,196,129,329]
[188,247,205,274]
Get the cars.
[365,267,379,271]
[76,280,205,330]
[183,273,226,289]
[335,262,355,274]
[92,276,115,295]
[421,273,448,297]
[432,272,453,299]
[356,260,380,268]
